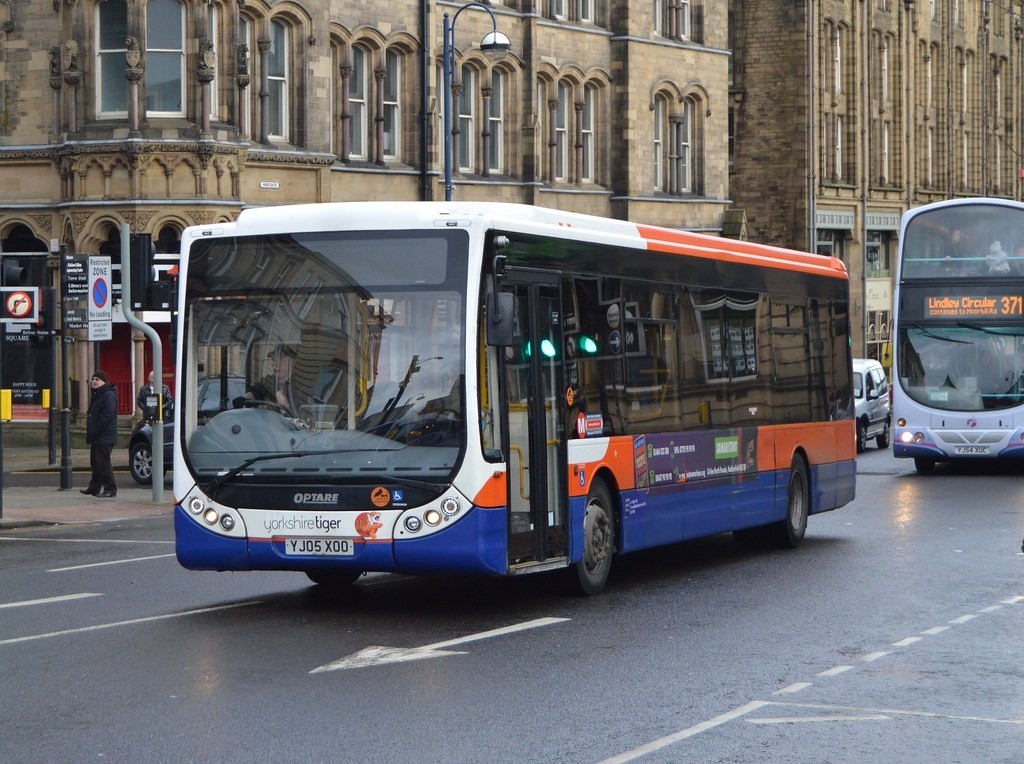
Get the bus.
[167,198,858,597]
[890,198,1024,479]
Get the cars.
[127,374,245,486]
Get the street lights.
[442,2,513,201]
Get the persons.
[137,370,172,421]
[922,343,1024,407]
[986,240,1024,276]
[860,375,874,395]
[232,345,317,423]
[939,225,976,269]
[79,371,118,497]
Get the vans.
[853,357,892,453]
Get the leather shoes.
[98,491,115,497]
[79,487,101,496]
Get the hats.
[990,241,1002,252]
[267,345,296,358]
[92,370,108,383]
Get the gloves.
[232,396,246,409]
